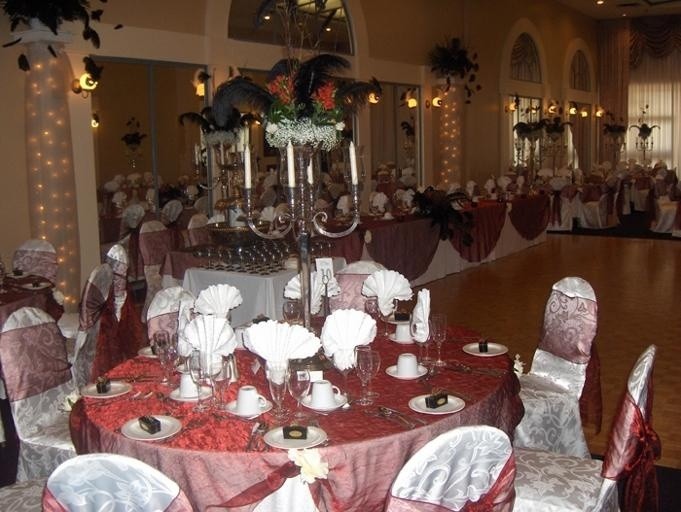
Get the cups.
[472,197,478,203]
[312,380,339,406]
[385,212,392,217]
[491,193,496,198]
[180,375,198,397]
[397,354,418,376]
[395,324,411,340]
[236,387,267,412]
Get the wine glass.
[361,350,381,399]
[156,331,170,385]
[281,302,300,324]
[160,342,180,389]
[379,302,398,336]
[410,314,436,376]
[211,361,226,423]
[268,367,289,424]
[194,238,337,276]
[430,315,447,368]
[189,350,209,412]
[355,344,373,407]
[288,371,310,420]
[366,300,378,335]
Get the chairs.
[513,344,660,511]
[41,454,192,512]
[59,264,131,386]
[511,279,602,460]
[0,307,77,483]
[384,425,516,512]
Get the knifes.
[246,420,259,453]
[379,405,428,426]
[244,422,261,452]
[112,376,158,379]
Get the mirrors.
[509,94,599,174]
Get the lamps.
[71,57,104,98]
[505,99,604,118]
[426,97,443,109]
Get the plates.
[462,343,510,356]
[301,395,348,416]
[225,399,273,419]
[264,426,328,449]
[22,282,53,290]
[8,271,31,279]
[170,385,214,401]
[82,382,134,398]
[121,415,182,441]
[390,334,416,344]
[409,394,466,414]
[385,364,428,381]
[380,216,395,220]
[385,311,416,326]
[138,346,160,359]
[490,197,498,200]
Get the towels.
[241,318,324,384]
[180,313,234,372]
[320,309,378,370]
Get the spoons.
[255,418,268,451]
[380,407,415,429]
